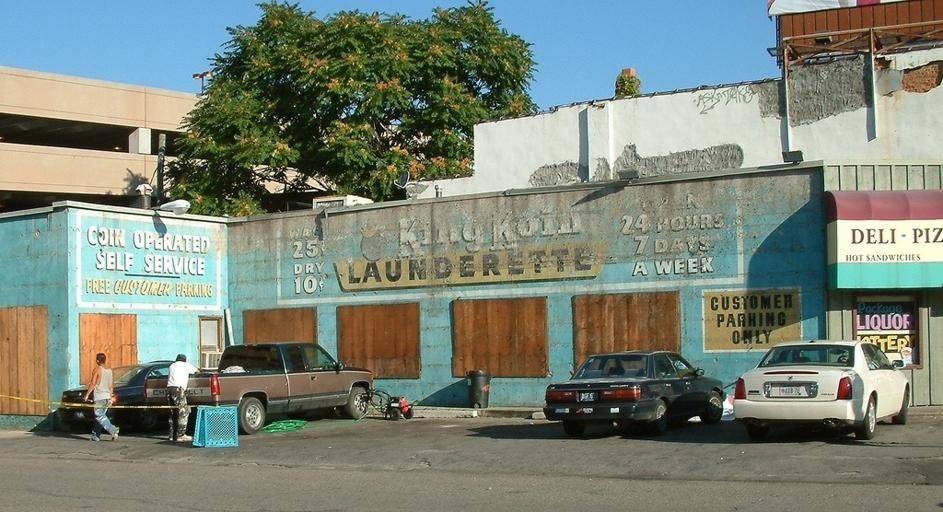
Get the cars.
[57,360,203,432]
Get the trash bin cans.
[467,370,489,408]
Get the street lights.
[135,183,191,215]
[192,71,211,93]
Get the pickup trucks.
[184,342,373,434]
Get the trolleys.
[360,390,419,421]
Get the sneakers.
[168,432,193,442]
[112,426,120,441]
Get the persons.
[82,351,120,442]
[166,354,201,443]
